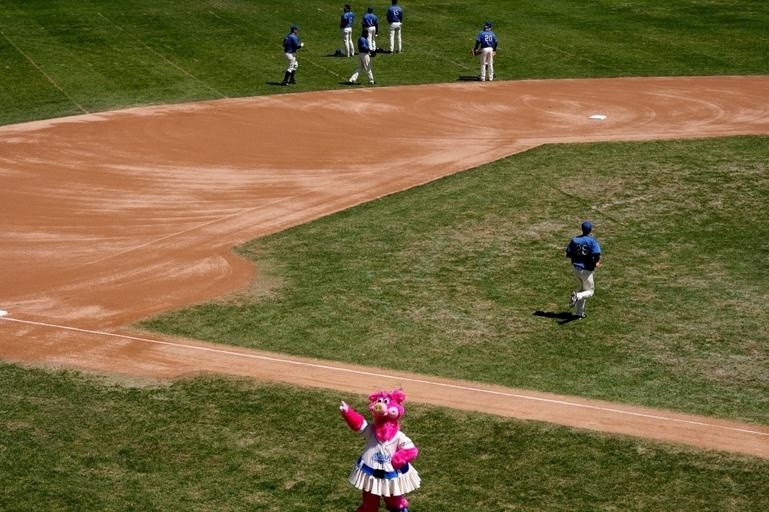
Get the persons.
[345,30,376,85]
[361,6,379,58]
[281,26,305,86]
[565,220,601,319]
[339,5,355,58]
[386,0,404,53]
[469,21,498,82]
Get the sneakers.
[288,81,295,84]
[569,291,577,307]
[577,312,587,320]
[282,82,289,86]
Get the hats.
[582,221,592,234]
[367,6,373,11]
[484,26,489,29]
[290,25,298,30]
[485,22,491,28]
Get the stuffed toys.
[339,385,422,512]
